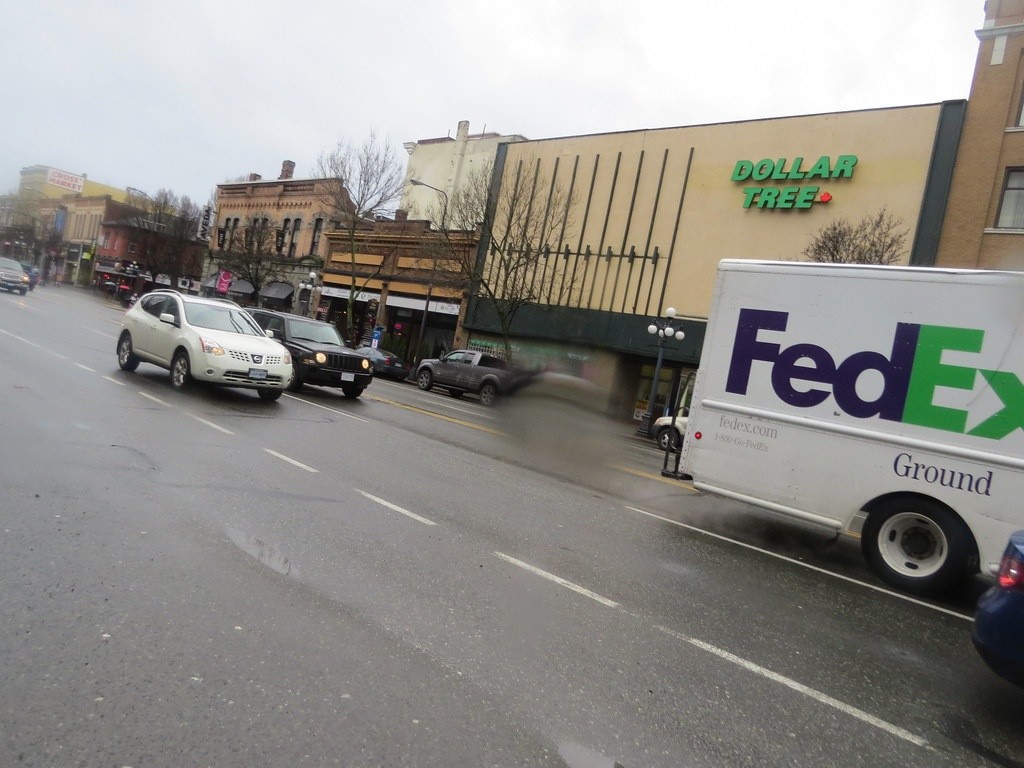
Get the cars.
[21,263,41,292]
[0,258,30,296]
[353,347,411,382]
[651,416,690,453]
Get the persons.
[106,286,113,299]
[32,266,40,274]
[130,293,139,304]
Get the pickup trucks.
[417,350,532,407]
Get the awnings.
[201,276,294,307]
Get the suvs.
[244,306,374,399]
[115,289,294,404]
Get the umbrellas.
[119,285,131,290]
[105,282,115,286]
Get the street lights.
[633,306,685,445]
[126,187,158,291]
[1,207,52,280]
[408,179,448,386]
[24,186,58,237]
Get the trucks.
[682,258,1024,596]
